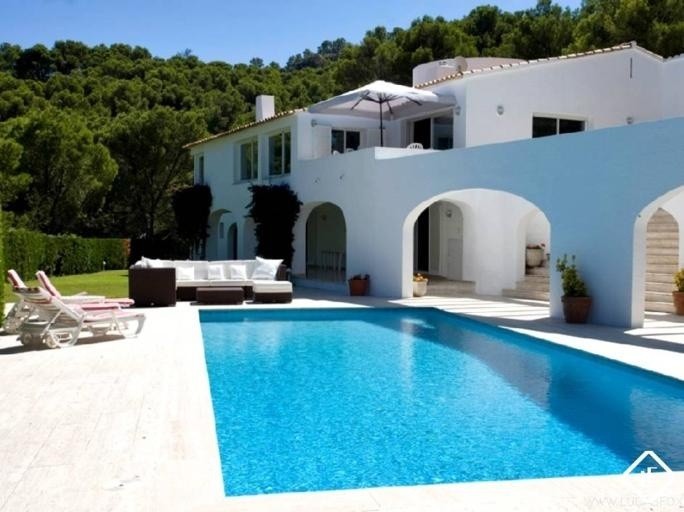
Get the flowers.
[672,268,684,291]
[554,253,585,296]
[412,273,426,281]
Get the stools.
[253,281,292,304]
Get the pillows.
[206,256,285,280]
[141,255,195,281]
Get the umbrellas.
[307,80,457,147]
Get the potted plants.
[348,273,371,296]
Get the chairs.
[2,268,145,349]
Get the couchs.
[128,259,286,305]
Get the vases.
[413,280,427,296]
[561,296,591,323]
[671,291,684,315]
